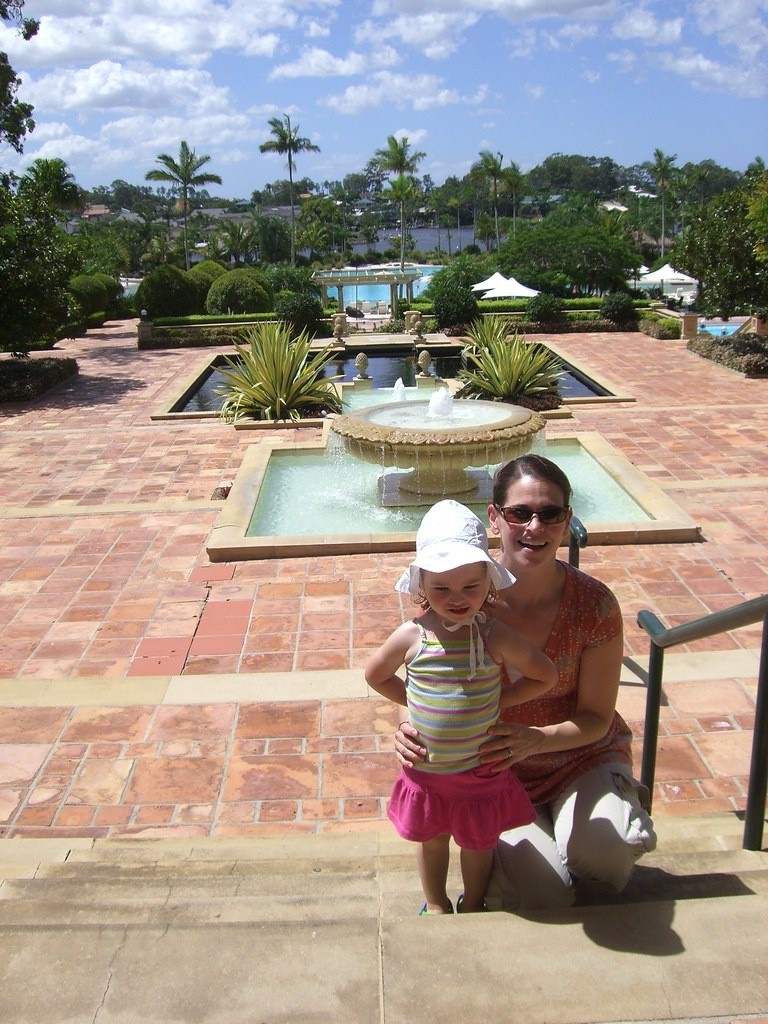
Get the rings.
[507,748,513,758]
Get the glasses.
[493,502,570,526]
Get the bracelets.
[398,721,409,730]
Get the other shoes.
[457,892,491,913]
[419,896,455,915]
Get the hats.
[394,499,518,597]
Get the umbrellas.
[469,272,543,301]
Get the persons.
[386,454,658,911]
[364,500,559,917]
